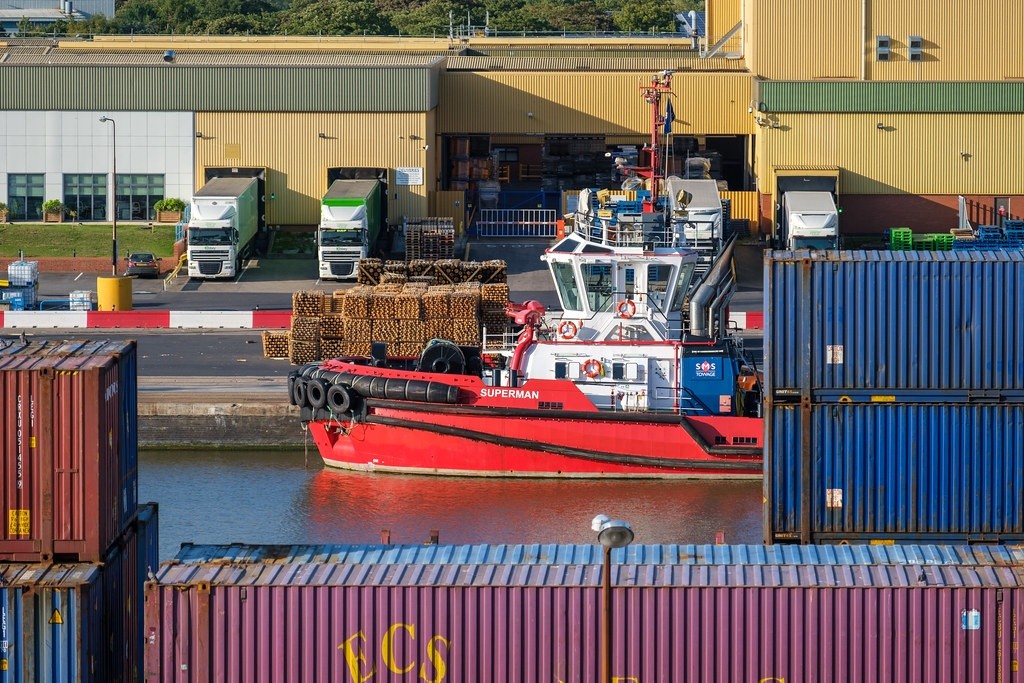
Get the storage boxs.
[0,246,1024,682]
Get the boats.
[289,69,765,478]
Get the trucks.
[188,176,260,280]
[783,190,841,251]
[668,179,724,278]
[315,178,383,281]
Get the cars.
[124,253,164,278]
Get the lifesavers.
[557,321,577,339]
[582,359,601,379]
[616,299,636,319]
